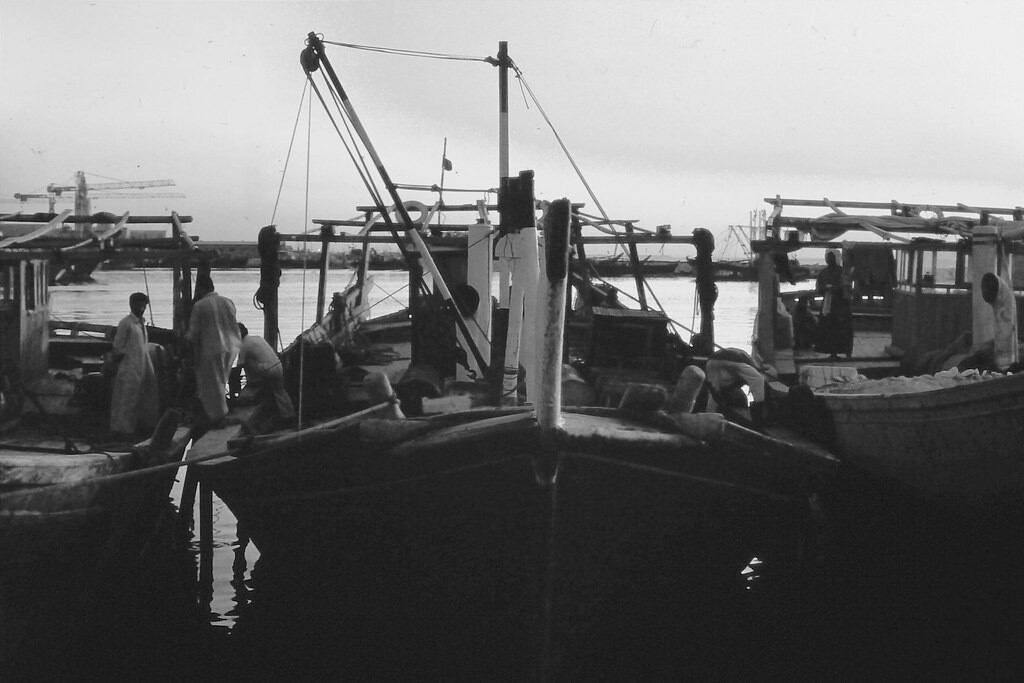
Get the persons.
[787,295,819,350]
[110,292,160,434]
[705,349,765,413]
[235,322,296,419]
[814,252,853,358]
[177,277,242,429]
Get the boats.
[1,32,1024,655]
